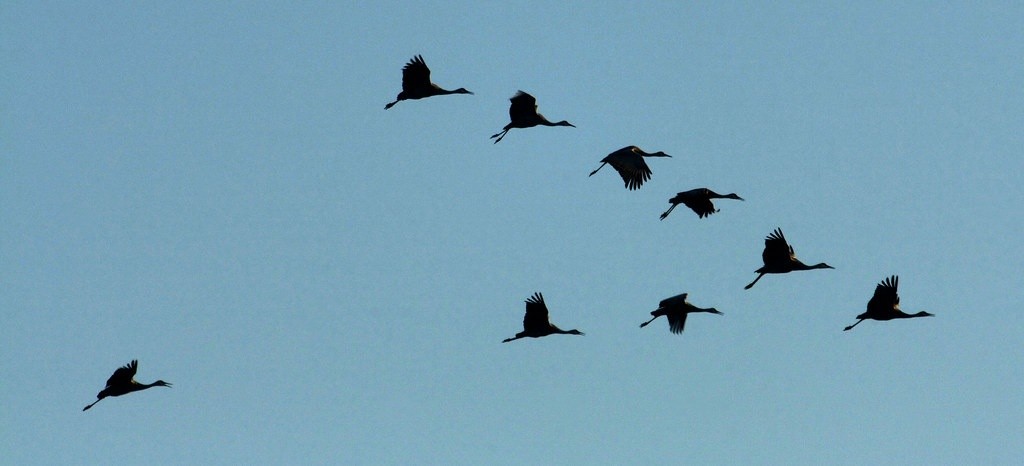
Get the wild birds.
[743,226,835,291]
[82,360,173,412]
[489,90,576,145]
[383,54,474,111]
[844,275,936,332]
[501,292,586,344]
[657,188,746,222]
[588,145,672,191]
[638,292,726,335]
